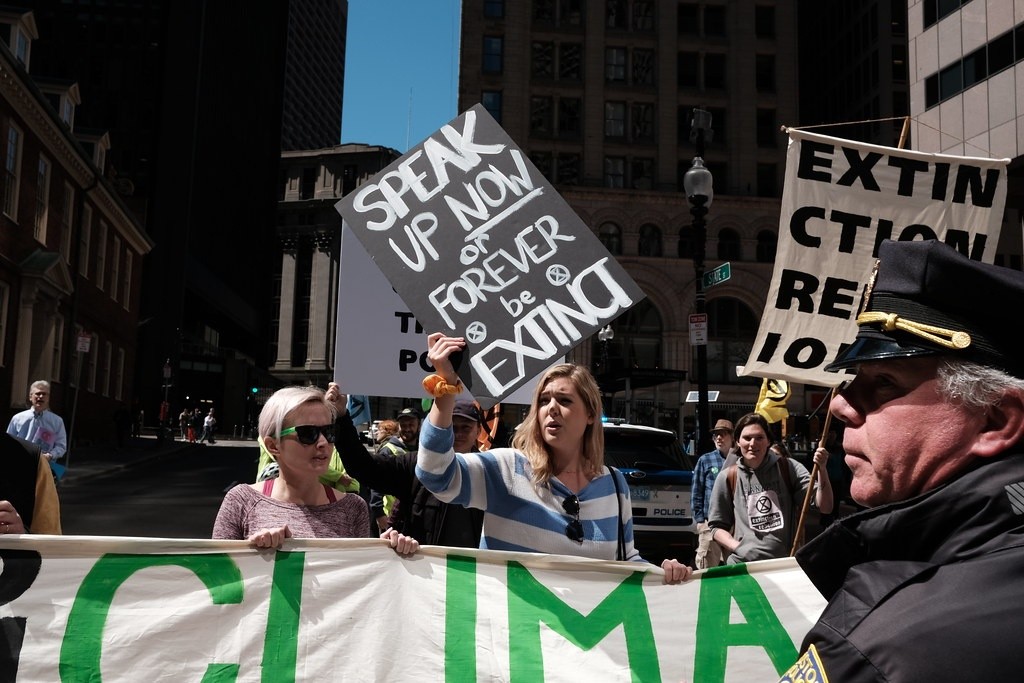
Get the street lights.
[683,155,718,462]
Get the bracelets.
[423,375,464,398]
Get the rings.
[411,537,416,542]
[6,524,9,534]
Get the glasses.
[712,430,728,438]
[562,494,584,545]
[272,423,341,445]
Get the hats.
[396,408,421,421]
[452,400,480,422]
[825,240,1023,380]
[710,419,734,434]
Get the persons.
[691,413,833,570]
[415,332,693,584]
[0,430,62,535]
[256,382,485,549]
[212,387,419,554]
[778,239,1024,683]
[6,380,66,485]
[160,408,217,443]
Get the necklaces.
[554,456,587,474]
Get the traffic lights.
[250,386,259,396]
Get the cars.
[579,418,706,536]
